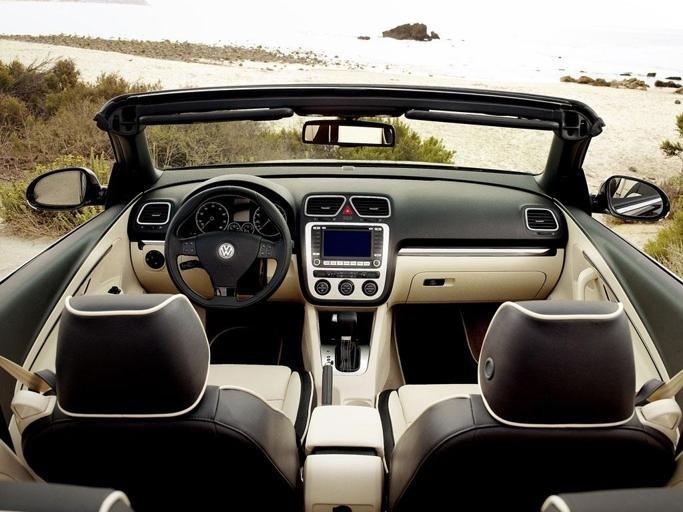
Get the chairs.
[375,296,682,512]
[9,293,316,512]
